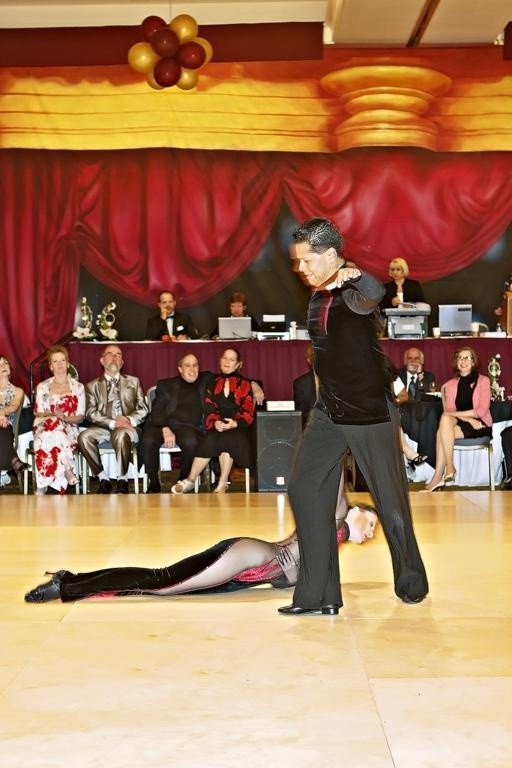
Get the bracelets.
[417,372,422,375]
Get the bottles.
[496,323,503,332]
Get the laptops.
[215,317,252,340]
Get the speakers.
[256,411,303,492]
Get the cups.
[288,326,296,340]
[432,327,441,339]
[469,323,480,338]
[162,335,170,342]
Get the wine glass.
[418,380,437,399]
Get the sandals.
[171,477,195,494]
[214,479,230,493]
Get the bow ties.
[166,315,174,319]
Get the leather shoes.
[11,457,31,475]
[402,592,426,604]
[65,466,80,486]
[98,477,161,494]
[278,599,339,616]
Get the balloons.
[128,15,212,90]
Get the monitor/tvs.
[439,304,472,336]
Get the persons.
[294,346,317,425]
[210,293,258,338]
[379,258,426,310]
[397,349,438,466]
[401,430,427,469]
[420,348,491,494]
[0,347,267,494]
[24,461,379,602]
[145,291,194,343]
[277,218,428,615]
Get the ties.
[408,376,416,401]
[107,378,122,420]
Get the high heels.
[423,479,445,491]
[444,469,457,482]
[408,454,433,472]
[24,570,67,603]
